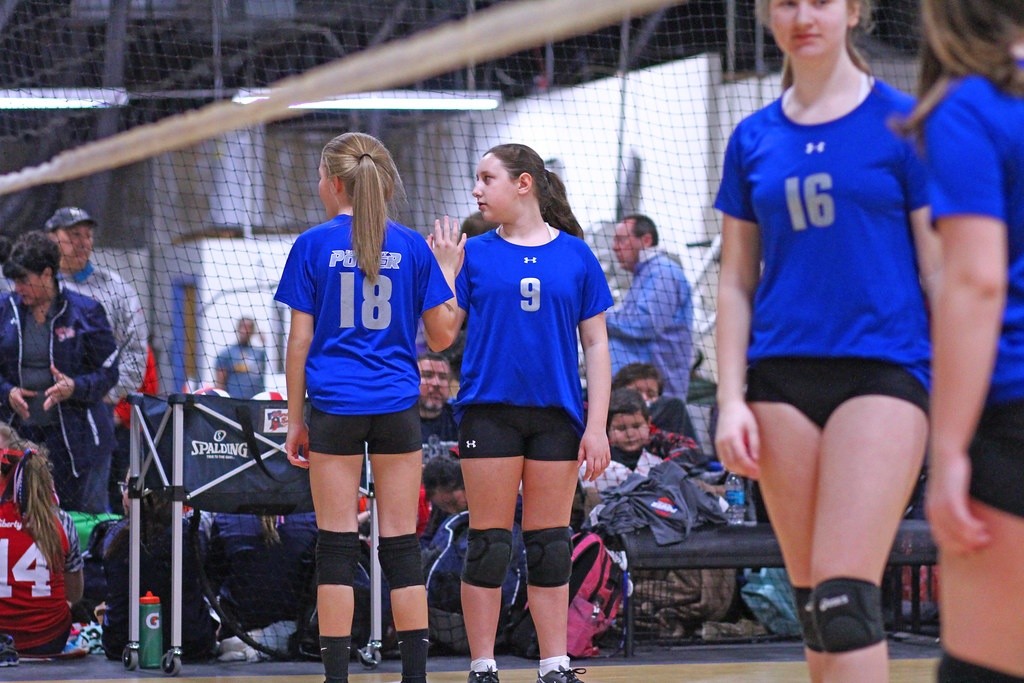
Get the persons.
[712,0,936,683]
[871,0,1024,683]
[0,132,693,683]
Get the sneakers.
[467,666,500,683]
[536,665,586,683]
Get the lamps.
[231,86,504,109]
[0,87,129,109]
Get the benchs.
[613,520,938,659]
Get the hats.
[44,207,99,233]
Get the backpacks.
[509,525,623,661]
[423,508,526,653]
[101,500,220,665]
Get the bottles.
[725,472,746,525]
[139,591,163,669]
